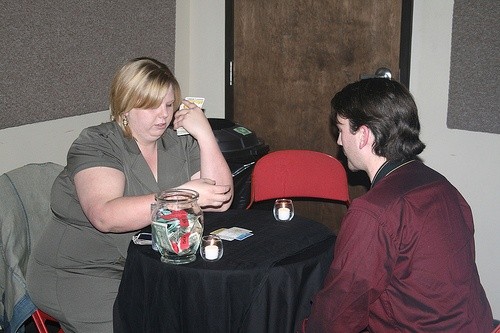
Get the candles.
[278,203,290,221]
[205,239,219,260]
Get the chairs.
[244,149,352,209]
[0,163,65,333]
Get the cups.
[273,200,294,222]
[200,235,223,262]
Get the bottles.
[151,189,204,264]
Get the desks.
[113,208,337,333]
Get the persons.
[25,58,234,333]
[303,79,494,333]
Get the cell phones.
[135,233,153,245]
